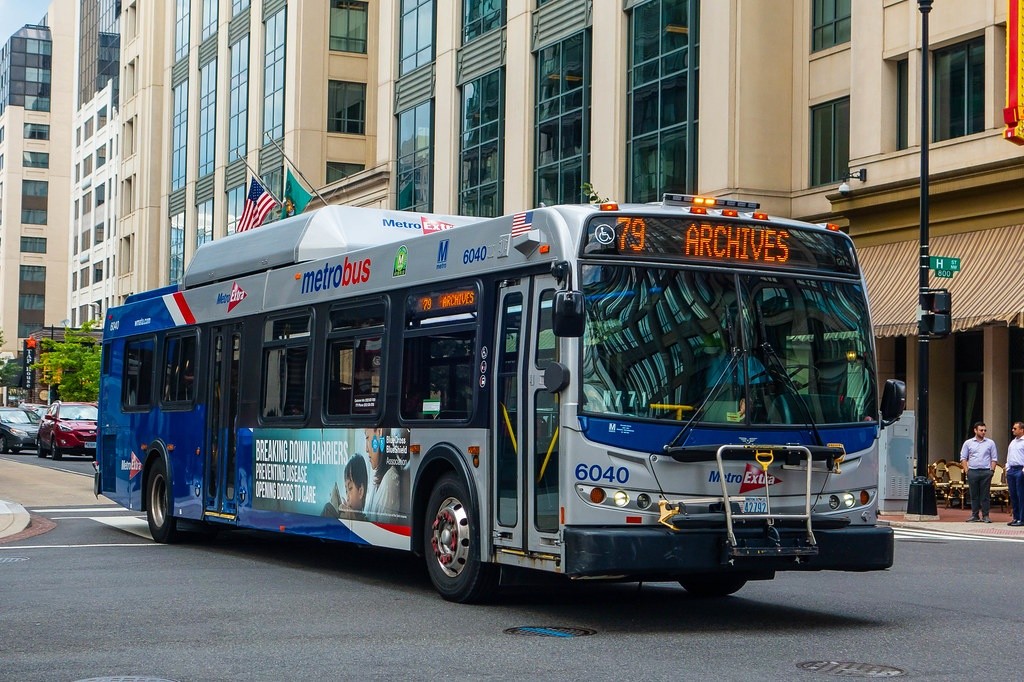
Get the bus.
[93,194,909,604]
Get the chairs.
[330,382,353,417]
[927,459,972,510]
[988,463,1010,514]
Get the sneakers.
[966,515,980,522]
[982,516,992,523]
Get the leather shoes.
[1007,520,1024,526]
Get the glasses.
[1012,427,1022,430]
[976,429,987,432]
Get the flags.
[279,165,315,219]
[236,176,278,232]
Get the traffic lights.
[919,291,953,336]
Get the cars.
[17,401,50,418]
[35,400,98,459]
[0,407,42,454]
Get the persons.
[1005,422,1024,526]
[707,298,772,383]
[960,422,998,523]
[320,427,401,524]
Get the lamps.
[837,169,867,197]
